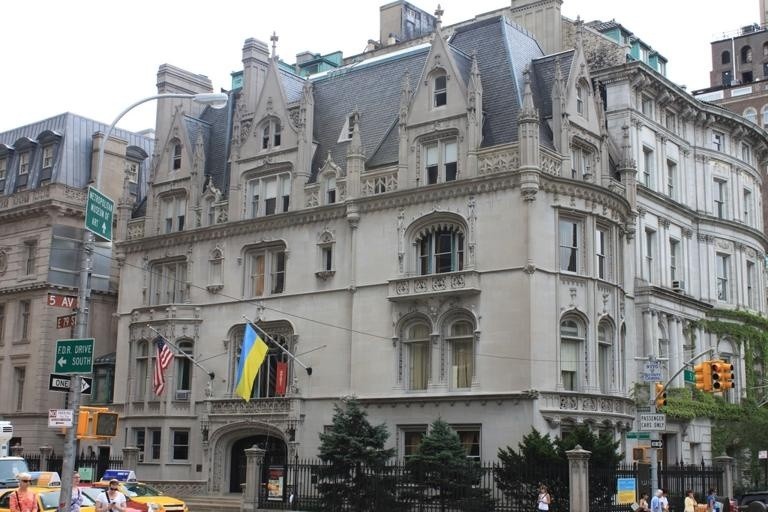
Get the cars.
[0,470,186,511]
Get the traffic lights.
[723,363,735,389]
[711,360,724,391]
[656,384,666,406]
[95,412,119,437]
[694,362,711,391]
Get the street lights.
[55,90,228,509]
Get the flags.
[152,333,176,398]
[234,321,268,402]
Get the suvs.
[740,491,768,511]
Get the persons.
[639,493,651,512]
[70,471,84,512]
[706,488,717,512]
[9,473,38,512]
[684,490,697,512]
[537,485,551,512]
[660,488,670,512]
[651,489,663,512]
[95,479,128,512]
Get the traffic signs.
[55,313,76,329]
[49,373,94,396]
[626,430,649,439]
[650,440,663,448]
[86,185,114,241]
[47,291,78,310]
[685,371,695,384]
[54,338,94,373]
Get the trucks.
[0,420,30,494]
